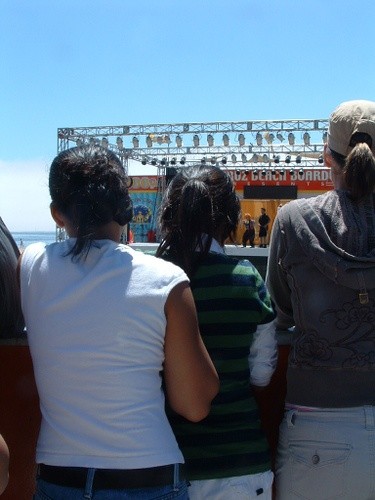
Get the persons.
[17,145,219,500]
[258,208,271,248]
[241,213,257,248]
[0,220,42,500]
[265,99,375,500]
[153,165,277,500]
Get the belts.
[34,462,183,491]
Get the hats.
[326,99,375,157]
